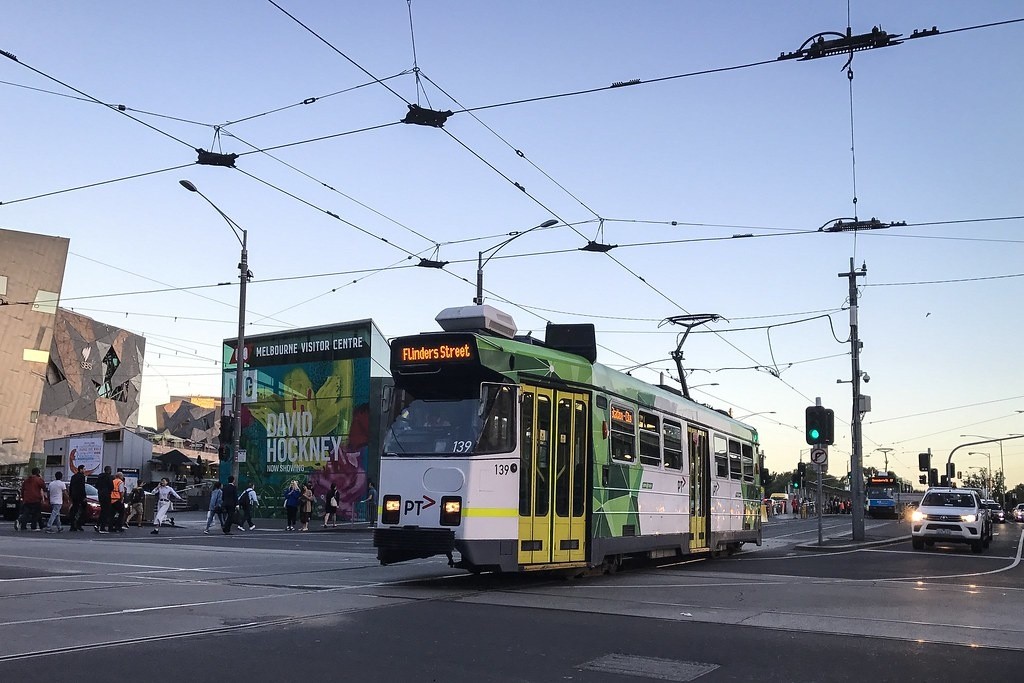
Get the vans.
[770,493,789,503]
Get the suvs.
[0,481,21,518]
[910,488,990,553]
[979,499,1005,523]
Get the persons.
[14,465,89,534]
[203,476,238,535]
[238,482,260,532]
[365,482,377,527]
[323,483,339,528]
[284,480,315,531]
[176,475,187,489]
[124,480,145,528]
[150,478,186,534]
[94,466,128,533]
[791,495,872,519]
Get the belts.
[160,499,169,502]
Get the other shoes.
[298,528,310,531]
[125,522,130,528]
[31,528,41,532]
[138,525,144,529]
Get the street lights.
[959,434,1005,511]
[178,179,247,491]
[969,452,991,491]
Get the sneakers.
[204,530,210,533]
[286,525,295,531]
[58,527,66,532]
[14,520,21,531]
[150,528,158,534]
[94,524,109,534]
[237,525,245,531]
[45,528,55,534]
[249,525,256,531]
[169,517,174,525]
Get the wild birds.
[926,312,931,317]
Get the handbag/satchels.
[231,505,245,525]
[331,490,337,507]
[214,504,221,514]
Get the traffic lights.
[919,475,926,484]
[806,406,826,445]
[793,474,800,489]
[848,472,851,479]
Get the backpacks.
[238,490,255,507]
[129,488,141,503]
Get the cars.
[36,482,130,526]
[1013,503,1024,522]
[764,499,775,505]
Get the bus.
[865,471,915,518]
[373,306,764,577]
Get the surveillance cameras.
[863,375,869,382]
